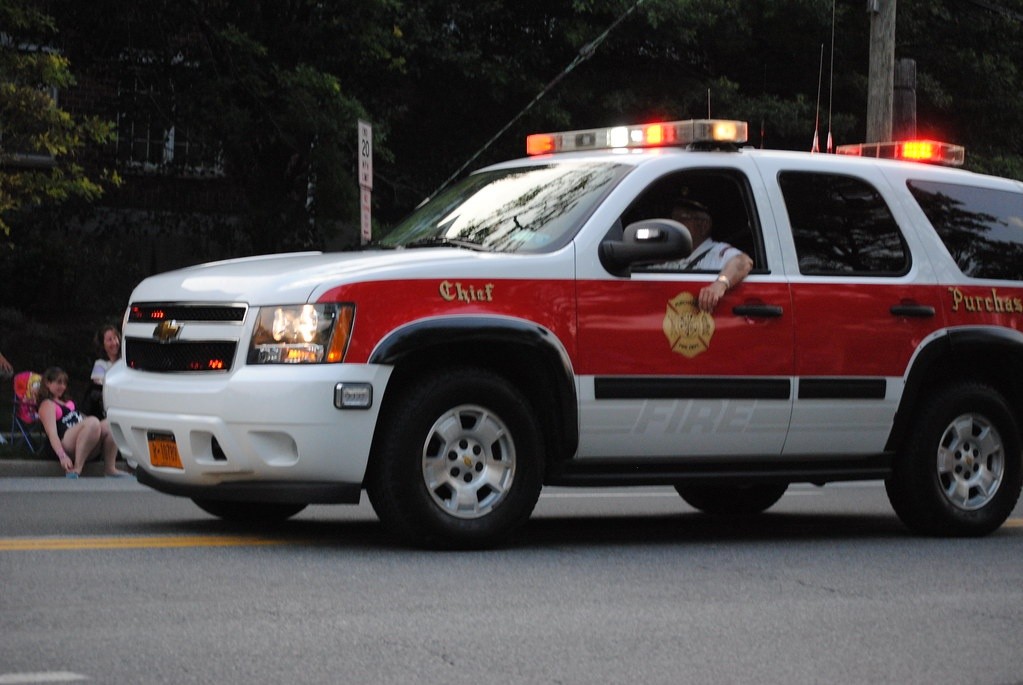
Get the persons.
[89,326,121,386]
[646,200,753,313]
[36,367,129,480]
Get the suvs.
[99,120,1022,550]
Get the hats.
[668,181,727,224]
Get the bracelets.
[715,275,730,290]
